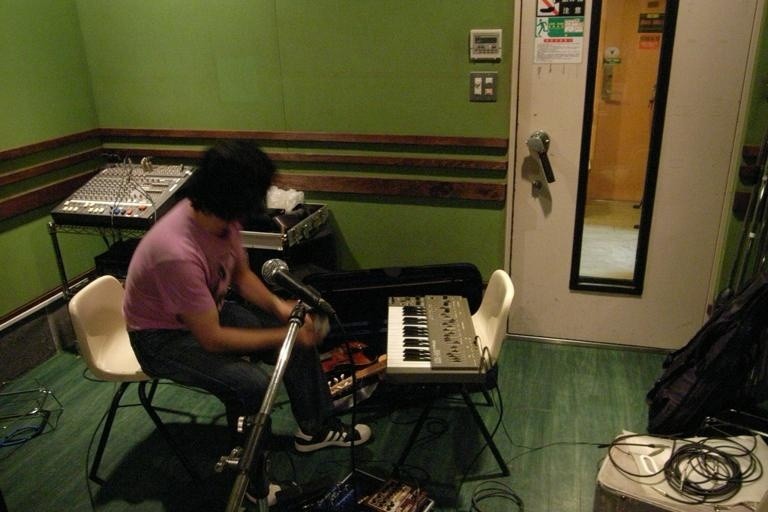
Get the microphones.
[261,258,337,317]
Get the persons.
[122,137,371,505]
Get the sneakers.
[246,482,281,507]
[294,418,371,452]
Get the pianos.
[387,294,487,384]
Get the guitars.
[320,341,377,376]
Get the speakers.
[592,433,768,512]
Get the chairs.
[65,275,239,481]
